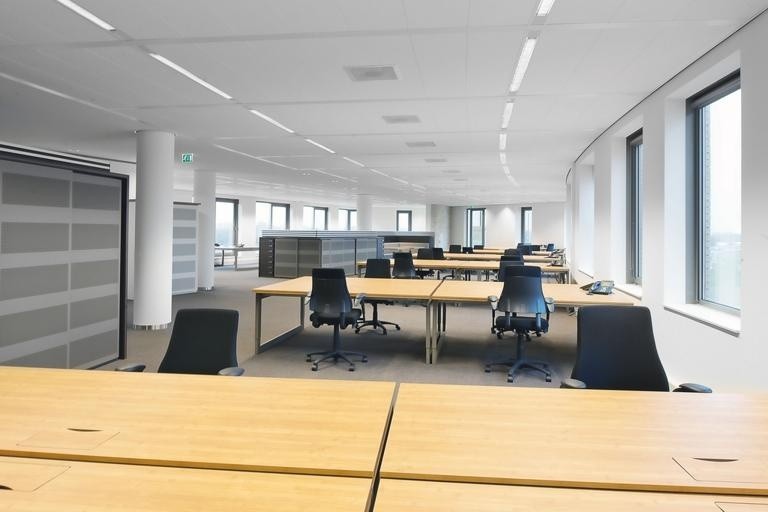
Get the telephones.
[587,280,614,295]
[551,259,563,266]
[549,252,559,258]
